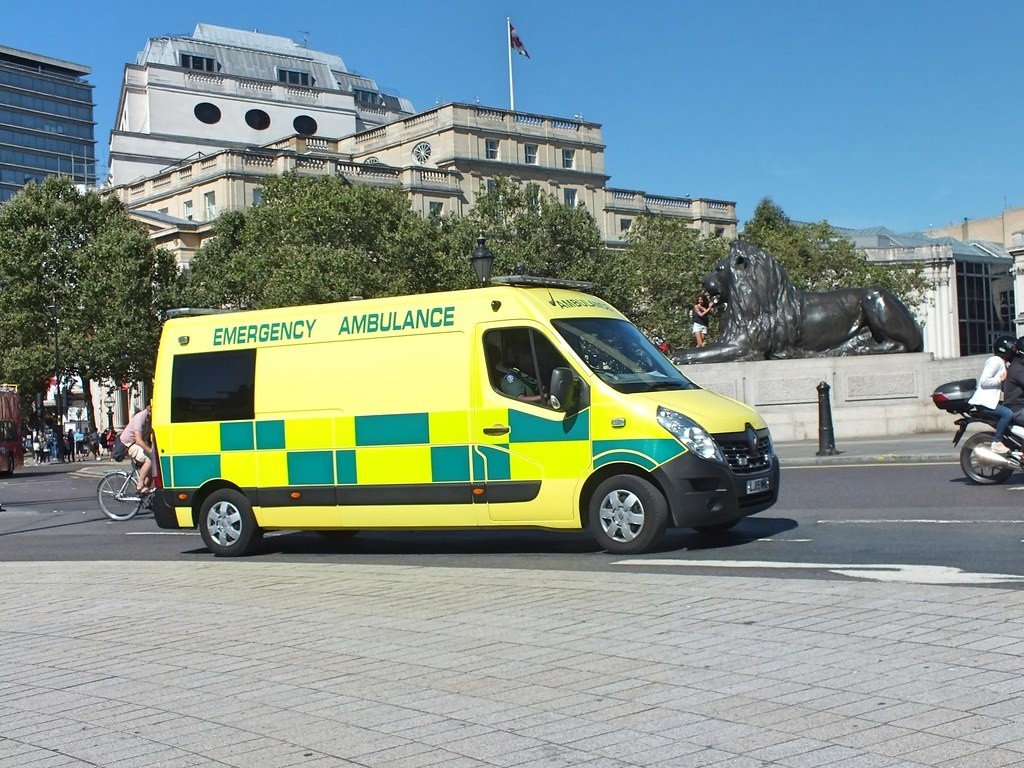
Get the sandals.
[134,485,150,494]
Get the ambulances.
[150,282,783,557]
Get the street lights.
[48,304,68,465]
[103,395,115,430]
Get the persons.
[1001,336,1024,428]
[643,323,669,355]
[119,396,158,496]
[968,335,1019,454]
[692,294,714,348]
[486,340,552,406]
[22,426,118,465]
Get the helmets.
[994,336,1018,361]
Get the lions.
[663,239,924,369]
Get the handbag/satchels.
[112,435,130,461]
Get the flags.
[509,24,532,60]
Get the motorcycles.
[932,378,1024,484]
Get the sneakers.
[990,443,1010,454]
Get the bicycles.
[96,459,154,522]
